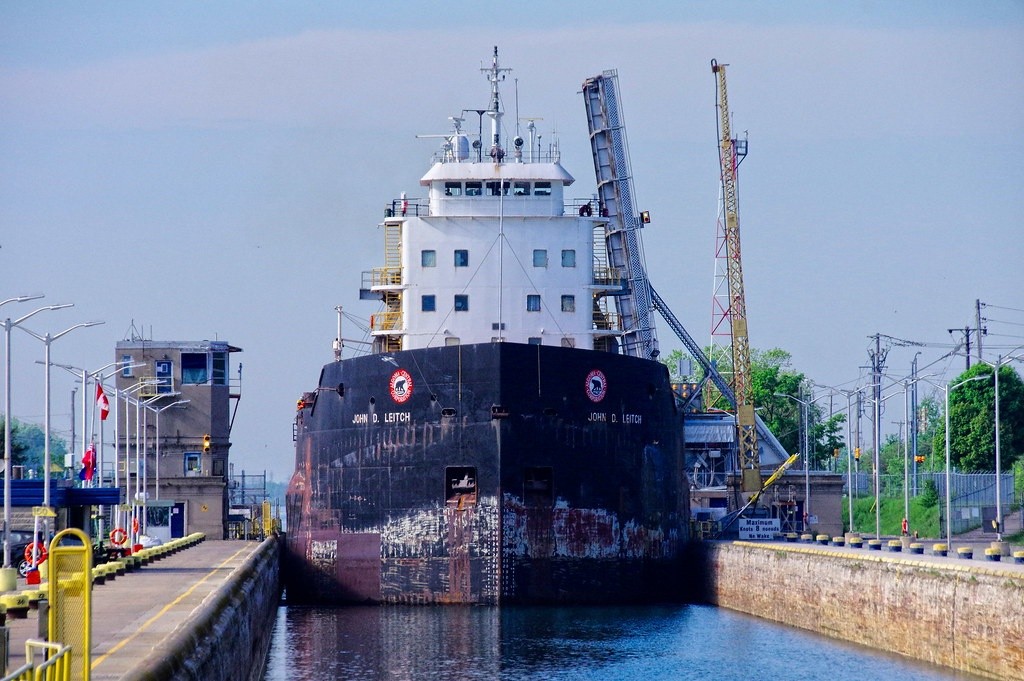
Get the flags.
[78,441,97,480]
[96,384,110,421]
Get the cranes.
[712,59,772,516]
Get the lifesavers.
[109,528,128,544]
[25,542,47,565]
[901,518,907,533]
[134,518,139,534]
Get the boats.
[283,45,692,604]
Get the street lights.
[35,360,192,556]
[905,376,992,552]
[0,294,46,305]
[841,389,906,539]
[14,322,107,580]
[955,352,1024,542]
[814,383,879,542]
[774,393,838,532]
[0,304,75,593]
[869,373,945,548]
